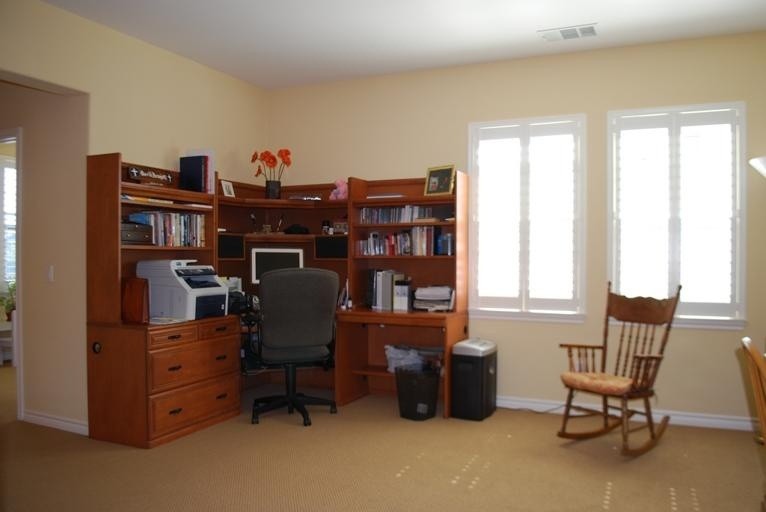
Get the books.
[185,204,212,208]
[182,151,216,194]
[121,195,173,204]
[355,226,452,256]
[365,270,453,311]
[180,153,205,194]
[359,206,440,225]
[129,213,205,247]
[204,155,208,193]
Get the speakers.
[218,235,245,260]
[316,236,348,258]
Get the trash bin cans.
[395,362,441,422]
[451,336,498,421]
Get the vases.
[266,181,281,199]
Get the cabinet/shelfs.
[216,169,468,419]
[86,152,240,449]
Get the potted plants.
[1,281,17,322]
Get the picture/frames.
[424,164,455,196]
[221,180,236,197]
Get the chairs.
[741,336,766,445]
[0,311,17,367]
[242,268,340,427]
[556,281,682,457]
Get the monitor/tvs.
[251,248,303,284]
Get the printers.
[136,259,228,320]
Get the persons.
[335,224,343,233]
[344,224,348,232]
[441,177,449,191]
[429,181,437,192]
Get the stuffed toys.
[329,179,347,199]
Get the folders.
[371,268,393,310]
[393,280,410,313]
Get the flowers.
[251,149,292,180]
